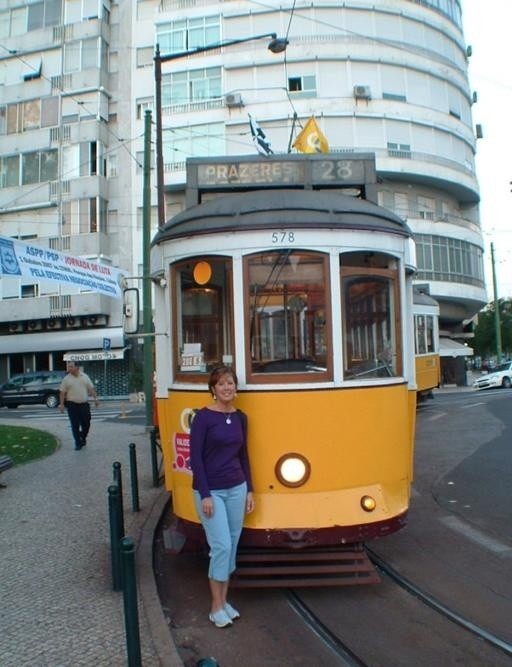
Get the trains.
[119,150,440,594]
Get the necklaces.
[216,402,234,424]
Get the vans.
[0,369,71,409]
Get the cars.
[473,360,512,391]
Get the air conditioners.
[46,319,61,329]
[87,315,107,326]
[66,317,81,327]
[26,320,42,331]
[225,93,242,106]
[354,85,371,98]
[8,322,23,332]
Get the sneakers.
[209,601,240,629]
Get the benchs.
[0,454,13,488]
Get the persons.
[57,360,102,450]
[189,364,253,626]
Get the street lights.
[152,30,290,232]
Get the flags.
[293,117,330,155]
[248,116,272,159]
